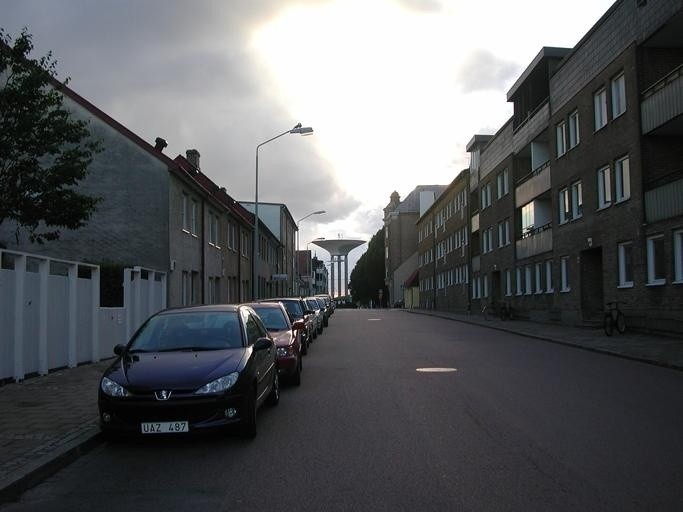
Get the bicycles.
[601,300,626,336]
[498,300,515,321]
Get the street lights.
[318,262,334,294]
[306,236,325,295]
[294,210,326,297]
[250,123,316,299]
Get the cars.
[393,298,404,309]
[341,302,358,308]
[98,294,337,448]
